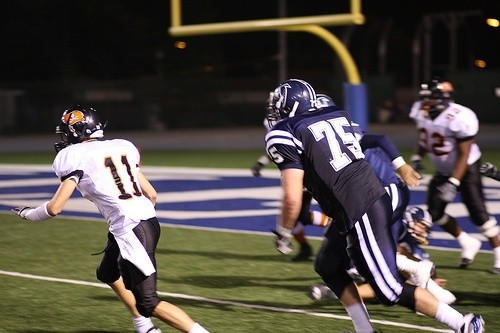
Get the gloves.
[10,201,55,222]
[438,177,461,201]
[271,228,293,256]
[411,154,424,170]
[479,162,497,179]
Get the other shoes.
[292,247,312,262]
[252,163,261,177]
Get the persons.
[250,79,500,333]
[11,105,209,333]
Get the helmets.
[54,105,104,153]
[419,79,453,118]
[316,94,336,109]
[402,206,433,245]
[265,79,315,128]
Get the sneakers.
[456,313,485,333]
[310,285,332,300]
[412,259,435,290]
[492,254,500,273]
[460,236,482,268]
